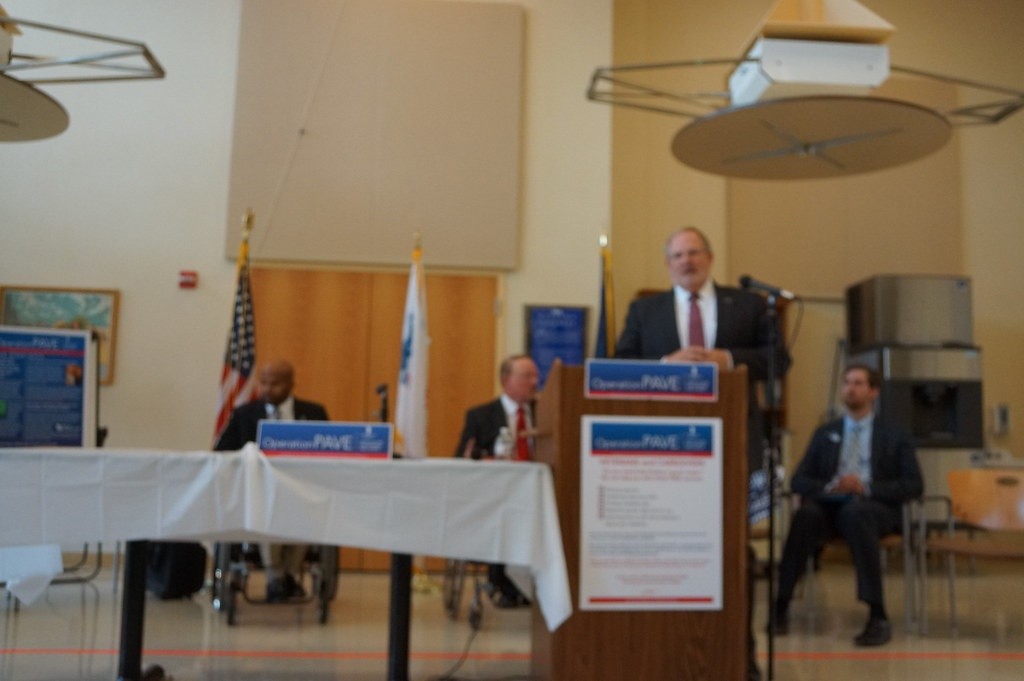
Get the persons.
[616,226,791,472]
[213,360,329,602]
[772,366,924,647]
[455,356,540,606]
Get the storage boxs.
[1,326,95,446]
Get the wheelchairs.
[209,539,340,627]
[439,552,519,622]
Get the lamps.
[583,0,1021,184]
[1,8,164,140]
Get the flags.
[216,241,259,435]
[394,257,427,457]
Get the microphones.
[739,274,801,301]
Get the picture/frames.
[1,283,120,386]
[521,303,590,394]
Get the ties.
[516,408,530,461]
[847,427,861,475]
[689,293,704,347]
[272,405,281,418]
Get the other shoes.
[854,620,888,646]
[262,565,305,604]
[773,616,789,634]
[490,587,517,607]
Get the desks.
[1,448,572,681]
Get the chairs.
[1,540,102,680]
[802,469,1023,637]
